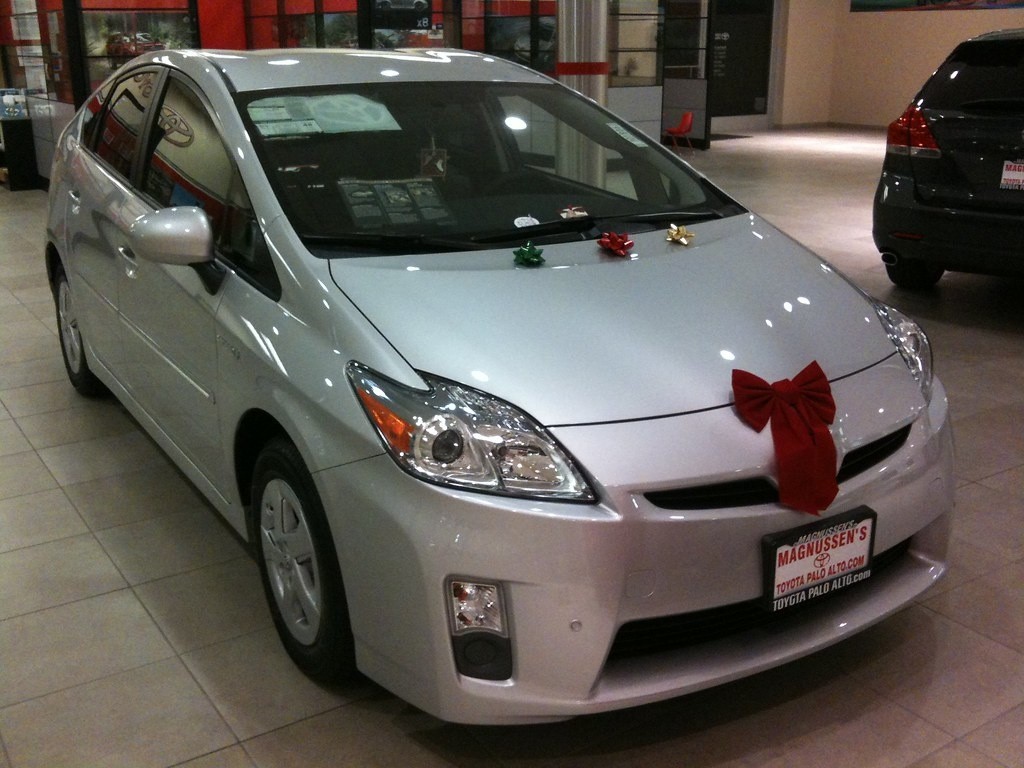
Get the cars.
[44,46,957,725]
[106,33,163,70]
[373,29,404,50]
[513,17,557,64]
[125,33,154,43]
[870,27,1024,294]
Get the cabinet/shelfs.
[0,118,42,192]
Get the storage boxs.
[0,167,9,183]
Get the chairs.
[411,102,523,234]
[664,111,695,157]
